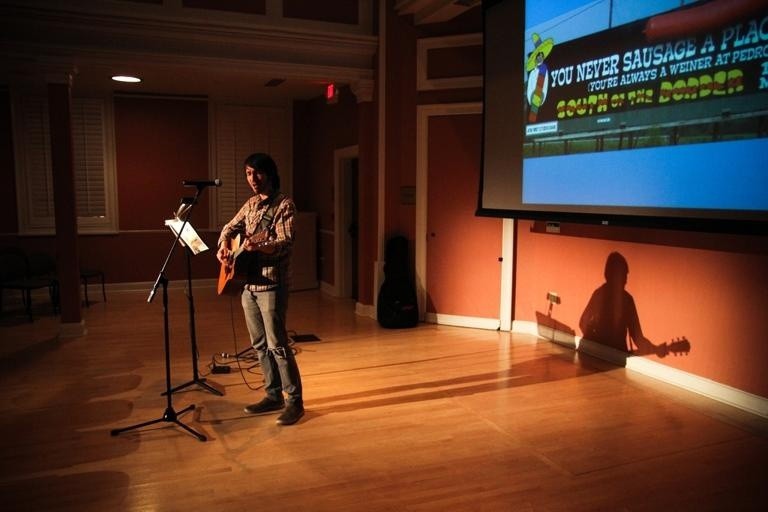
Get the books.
[171,219,211,258]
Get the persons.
[213,152,308,426]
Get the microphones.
[183,178,222,187]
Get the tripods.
[160,246,225,398]
[110,190,208,443]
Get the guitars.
[217,227,277,297]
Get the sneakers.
[276,402,306,425]
[242,396,285,415]
